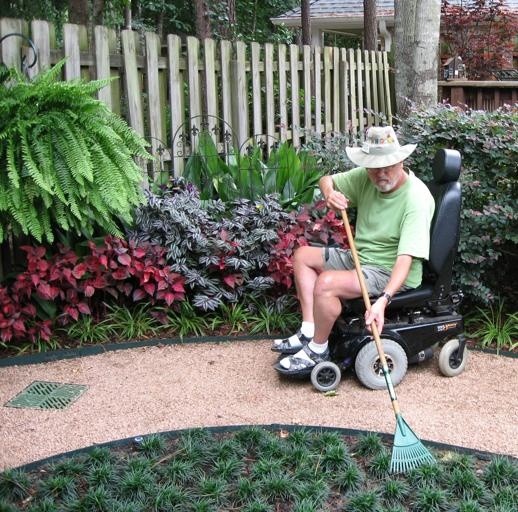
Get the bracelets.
[377,290,393,306]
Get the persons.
[271,124,437,377]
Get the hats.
[344,125,419,171]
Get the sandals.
[274,344,331,374]
[270,330,312,353]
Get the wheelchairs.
[277,149,468,391]
